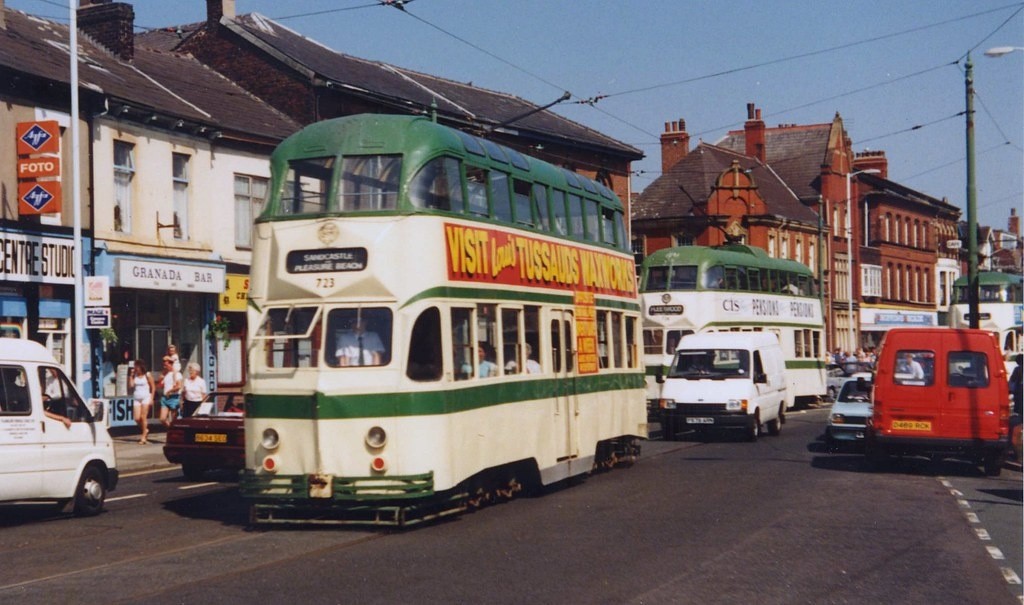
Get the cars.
[162,390,246,479]
[821,356,915,443]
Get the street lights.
[845,167,883,357]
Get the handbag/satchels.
[144,373,152,394]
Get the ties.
[357,336,364,366]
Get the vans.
[652,332,788,441]
[0,334,121,517]
[855,326,1010,476]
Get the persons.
[900,351,926,380]
[161,343,183,386]
[504,343,542,376]
[782,279,806,296]
[158,356,184,429]
[128,357,156,445]
[42,396,72,429]
[460,341,499,379]
[44,368,71,398]
[179,362,209,418]
[825,345,878,371]
[1008,352,1023,415]
[334,314,386,367]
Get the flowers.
[209,315,230,332]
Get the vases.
[215,330,225,339]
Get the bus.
[241,91,653,530]
[947,268,1023,381]
[638,176,828,422]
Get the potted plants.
[99,328,118,352]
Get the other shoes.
[138,440,148,444]
[141,429,148,439]
